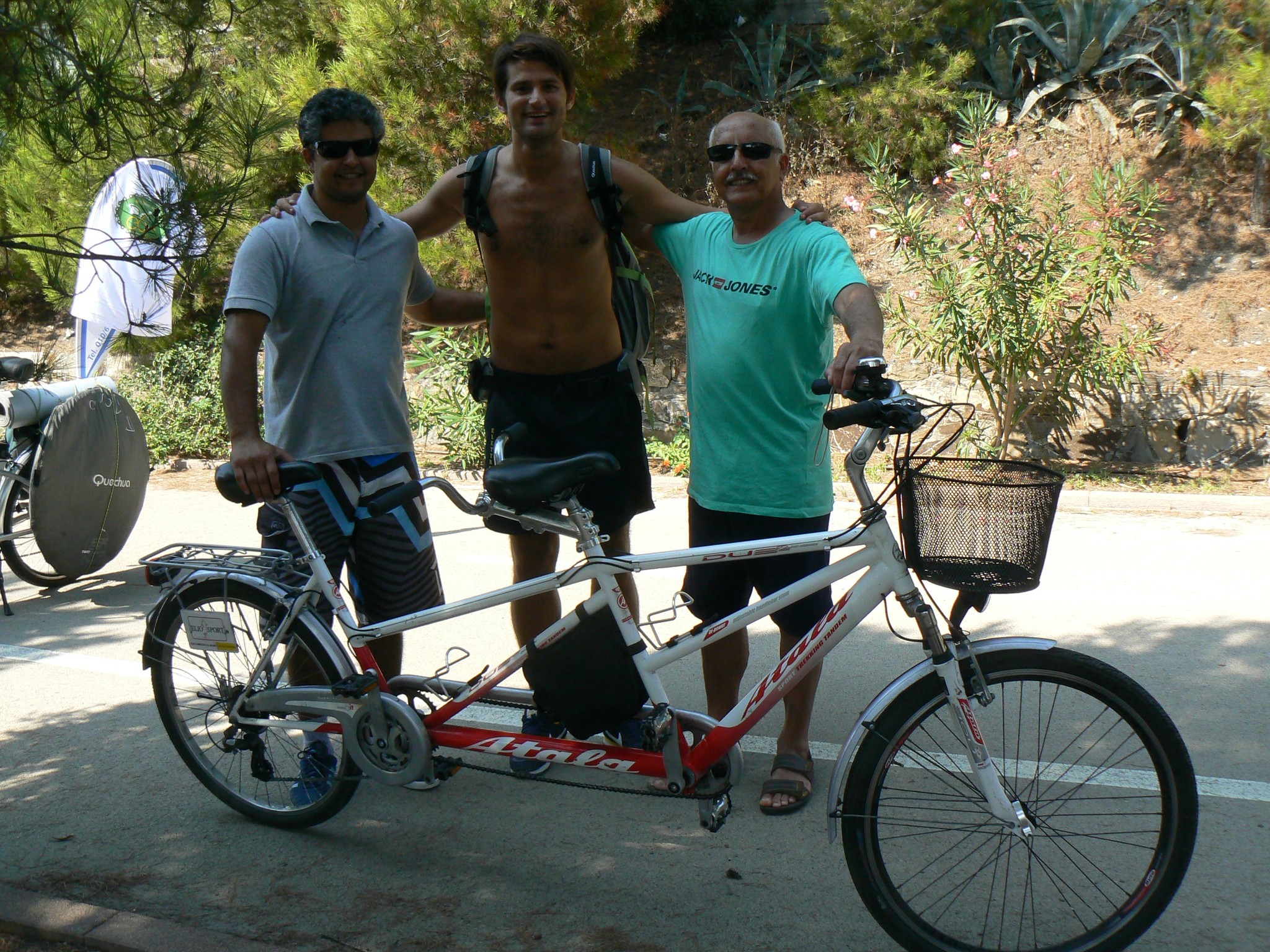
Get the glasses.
[308,138,378,158]
[707,143,783,162]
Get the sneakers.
[603,716,643,749]
[362,772,440,789]
[290,740,338,808]
[510,706,568,775]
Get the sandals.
[759,749,814,815]
[647,742,741,796]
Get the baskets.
[896,456,1066,594]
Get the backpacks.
[461,143,658,430]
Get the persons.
[216,84,487,808]
[622,110,885,817]
[262,28,837,787]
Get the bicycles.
[137,350,1202,952]
[0,355,121,618]
